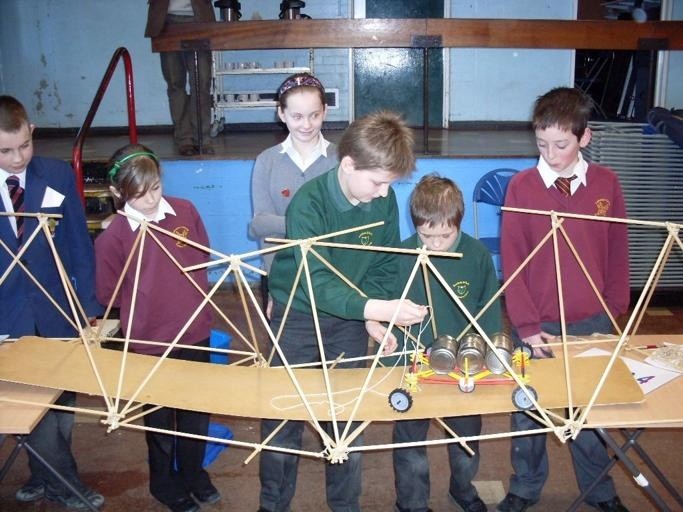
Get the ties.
[6,173,25,252]
[554,175,578,199]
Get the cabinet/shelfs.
[211,47,316,138]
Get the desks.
[547,334,683,512]
[0,319,121,512]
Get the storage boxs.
[210,329,232,365]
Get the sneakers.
[582,492,629,511]
[447,485,489,512]
[15,479,45,501]
[494,492,541,511]
[393,499,435,512]
[44,479,106,511]
[147,474,221,512]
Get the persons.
[257,108,427,512]
[0,95,105,512]
[94,144,221,512]
[145,0,215,158]
[247,74,339,322]
[496,88,631,512]
[373,172,502,512]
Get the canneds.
[484,333,512,374]
[429,334,459,376]
[457,333,487,375]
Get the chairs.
[473,168,519,289]
[575,52,612,121]
